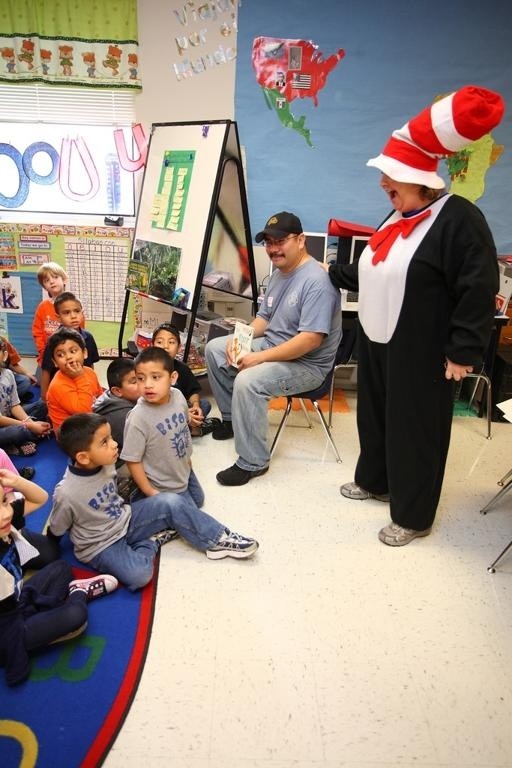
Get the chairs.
[481,467,511,572]
[454,354,495,441]
[271,316,359,466]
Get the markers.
[127,286,162,302]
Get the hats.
[256,212,302,243]
[365,86,505,190]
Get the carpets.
[0,437,161,767]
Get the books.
[230,322,254,371]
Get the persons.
[319,172,500,547]
[1,263,260,687]
[203,212,343,487]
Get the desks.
[336,308,509,419]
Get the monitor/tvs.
[269,232,328,277]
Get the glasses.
[262,236,296,247]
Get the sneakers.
[206,528,259,560]
[19,467,35,479]
[4,441,38,456]
[69,574,119,599]
[379,522,432,546]
[341,482,390,502]
[149,527,178,546]
[214,419,233,438]
[217,463,269,485]
[200,417,220,437]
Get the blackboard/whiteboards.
[125,118,231,311]
